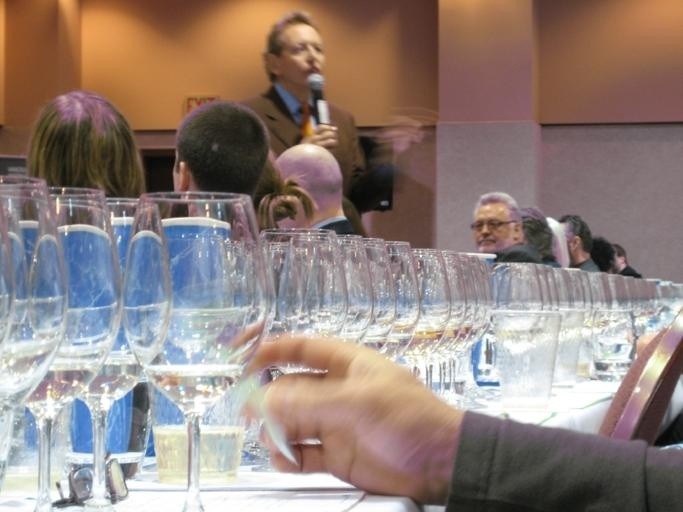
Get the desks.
[0,329,682,512]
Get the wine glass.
[2,172,682,511]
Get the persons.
[240,12,371,238]
[256,157,317,232]
[344,198,375,238]
[270,141,351,236]
[472,190,644,278]
[234,322,263,363]
[245,329,683,511]
[22,90,147,215]
[172,99,270,227]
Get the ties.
[299,104,314,137]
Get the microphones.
[306,73,330,126]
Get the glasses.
[467,219,518,231]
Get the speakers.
[345,135,393,210]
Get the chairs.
[593,297,683,447]
